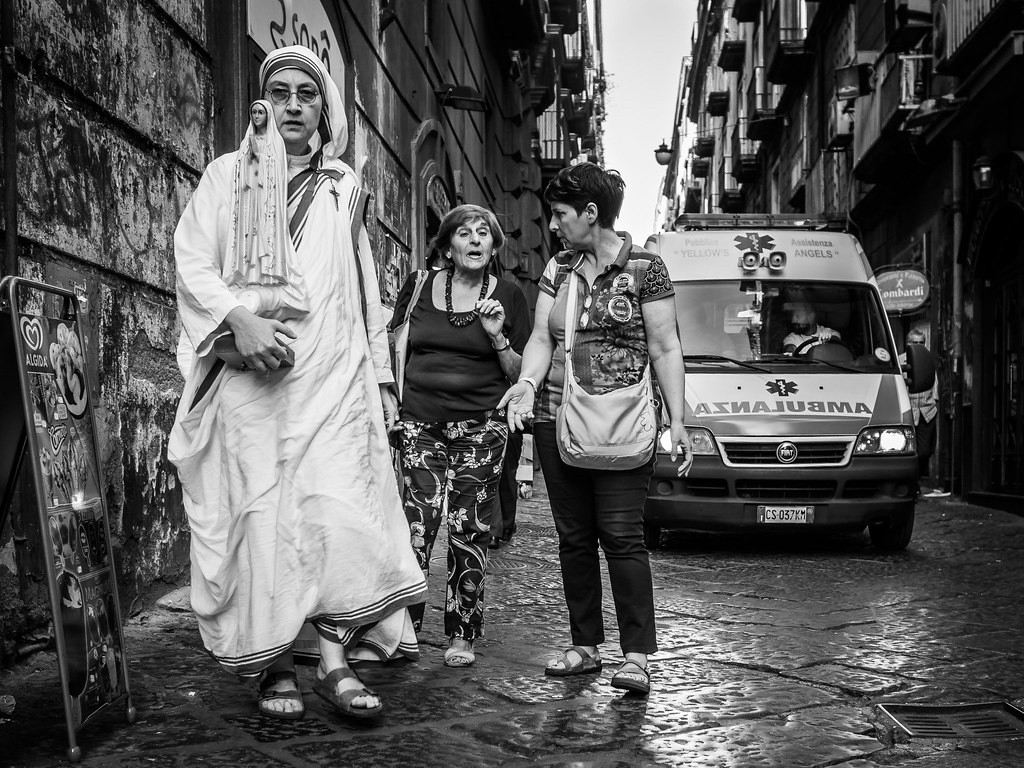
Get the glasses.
[909,341,925,344]
[264,87,320,105]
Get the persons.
[497,161,694,694]
[898,329,940,504]
[390,204,531,673]
[172,46,433,722]
[488,426,523,549]
[220,99,311,323]
[783,310,841,356]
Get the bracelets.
[517,378,537,392]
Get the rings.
[500,304,501,306]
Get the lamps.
[434,84,487,114]
[973,154,996,191]
[654,139,675,164]
[834,53,877,102]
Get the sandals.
[444,637,476,666]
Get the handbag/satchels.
[385,269,429,403]
[556,360,657,471]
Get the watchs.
[492,338,510,352]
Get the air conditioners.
[827,100,849,144]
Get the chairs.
[759,296,829,353]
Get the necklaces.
[444,264,490,326]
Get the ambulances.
[636,212,935,552]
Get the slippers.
[311,667,383,717]
[259,670,306,720]
[544,648,602,678]
[610,659,651,692]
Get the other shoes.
[488,536,499,549]
[918,477,933,494]
[500,527,514,541]
